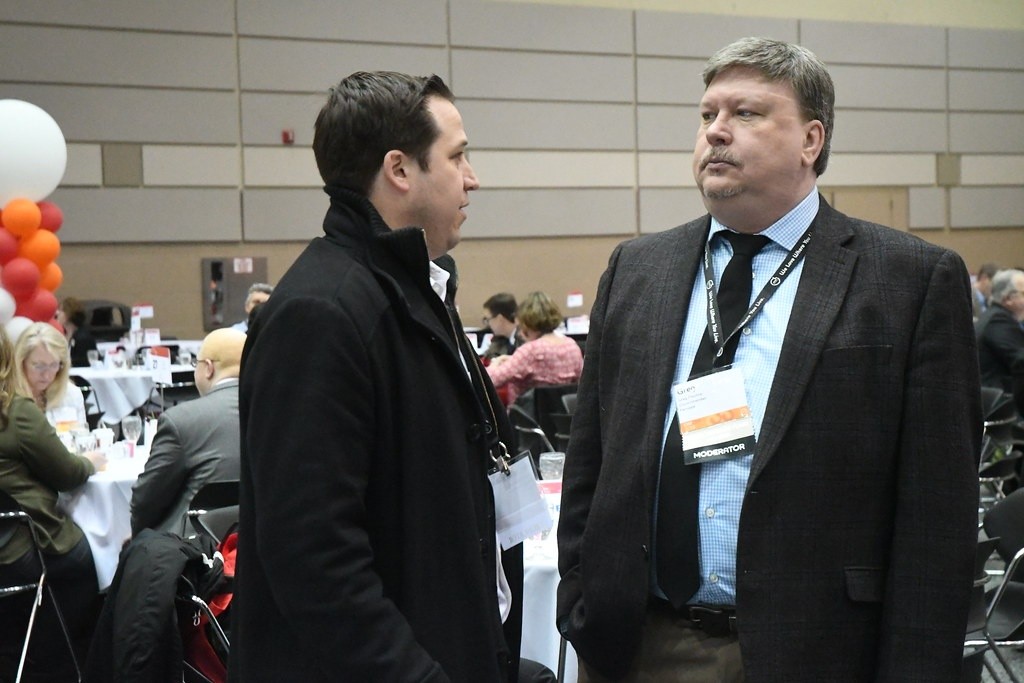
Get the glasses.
[26,359,60,371]
[190,358,214,368]
[483,314,497,327]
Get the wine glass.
[88,350,100,375]
[121,416,143,464]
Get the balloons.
[0,199,65,347]
[0,98,67,210]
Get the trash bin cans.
[69,298,132,387]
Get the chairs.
[1,333,1023,683]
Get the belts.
[647,598,739,637]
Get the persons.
[971,265,1024,499]
[481,291,583,416]
[229,66,524,683]
[232,283,274,331]
[555,37,984,683]
[15,322,89,435]
[57,295,104,367]
[130,328,247,539]
[0,321,108,674]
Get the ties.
[655,233,770,612]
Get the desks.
[54,442,152,597]
[64,365,154,440]
[97,339,204,366]
[508,478,580,683]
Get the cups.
[75,427,116,461]
[539,451,566,481]
